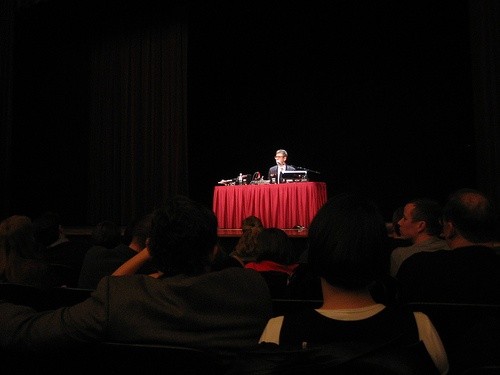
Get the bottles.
[239,173,242,185]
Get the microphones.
[276,160,280,165]
[236,173,252,179]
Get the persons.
[258,192,449,371]
[0,188,500,375]
[268,149,296,182]
[0,197,276,352]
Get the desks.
[213,181,328,235]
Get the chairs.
[0,279,500,375]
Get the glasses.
[274,156,285,159]
